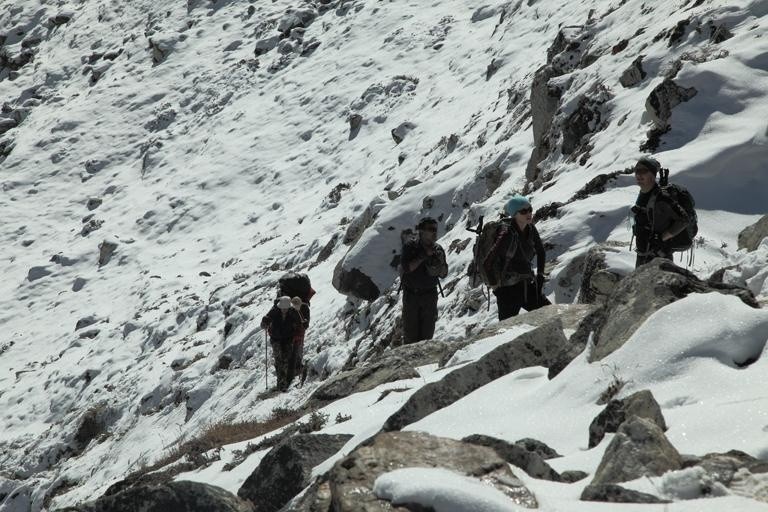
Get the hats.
[633,156,663,172]
[416,216,439,229]
[502,195,532,219]
[277,296,293,310]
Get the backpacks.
[278,270,311,328]
[467,219,509,292]
[658,181,700,255]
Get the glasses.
[517,205,534,216]
[422,226,438,233]
[633,166,650,176]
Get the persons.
[289,295,311,369]
[466,194,553,323]
[259,296,300,391]
[401,217,449,345]
[629,156,691,267]
